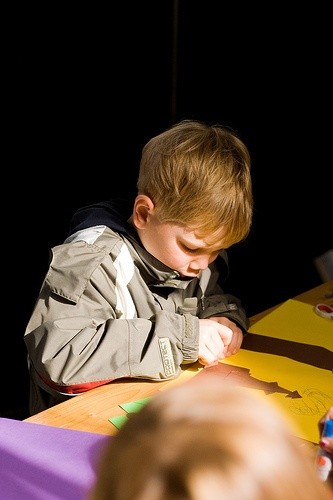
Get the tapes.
[315,303,333,319]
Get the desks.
[20,280,333,445]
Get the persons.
[24,119,253,416]
[89,383,332,500]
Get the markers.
[316,420,332,460]
[312,455,332,483]
